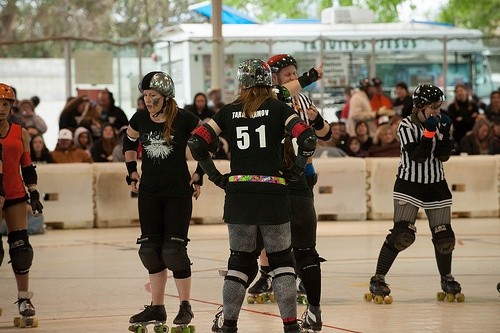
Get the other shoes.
[283,324,303,333]
[299,305,322,333]
[211,306,238,333]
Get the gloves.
[424,115,438,132]
[283,164,306,183]
[215,173,228,188]
[440,114,452,134]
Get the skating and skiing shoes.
[171,301,195,333]
[294,278,308,304]
[364,274,392,304]
[13,290,37,328]
[247,266,276,303]
[437,273,464,302]
[129,301,168,333]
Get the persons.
[122,71,218,333]
[364,83,465,304]
[0,83,43,327]
[187,59,316,333]
[0,79,500,164]
[247,54,332,303]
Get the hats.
[57,129,73,140]
[372,78,383,85]
[360,78,374,87]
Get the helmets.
[267,53,298,72]
[0,83,15,102]
[413,84,445,108]
[141,71,175,98]
[237,59,272,89]
